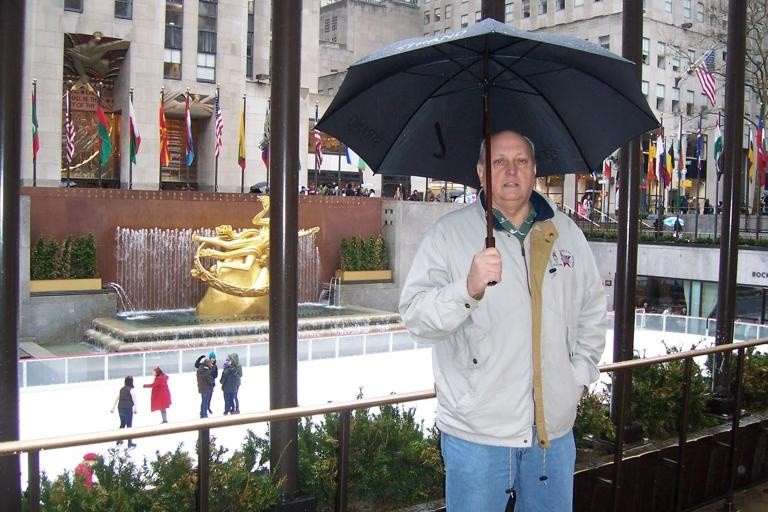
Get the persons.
[398,132,610,511]
[635,302,687,315]
[190,197,321,274]
[582,190,768,241]
[298,183,477,204]
[75,351,243,488]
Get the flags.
[236,99,247,169]
[257,108,270,169]
[159,103,173,167]
[344,145,370,173]
[590,112,768,183]
[30,83,40,161]
[214,99,224,157]
[95,92,114,169]
[65,90,77,164]
[183,99,194,166]
[696,50,717,107]
[315,117,324,173]
[128,93,141,165]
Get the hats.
[203,352,232,368]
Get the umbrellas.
[310,17,663,284]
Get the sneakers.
[198,408,240,418]
[128,442,136,447]
[117,441,122,445]
